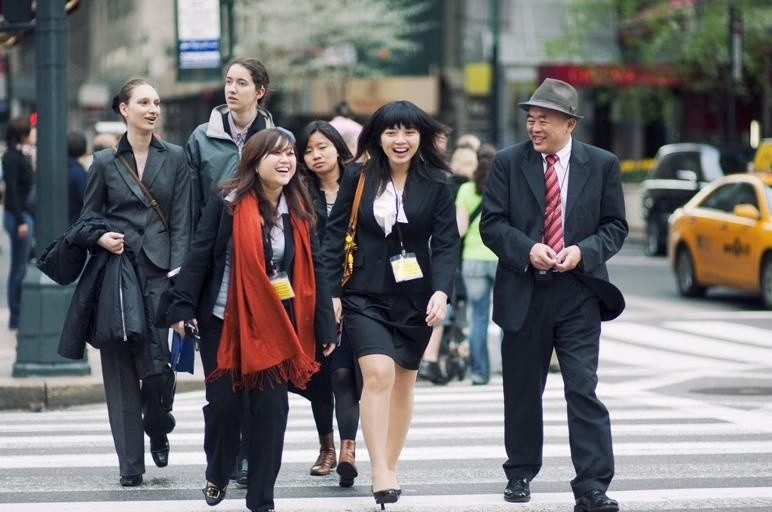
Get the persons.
[2,116,35,328]
[185,53,282,490]
[333,117,364,156]
[320,99,466,507]
[77,73,193,488]
[154,125,342,512]
[68,130,116,225]
[477,73,630,512]
[418,132,499,386]
[288,113,361,488]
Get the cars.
[639,136,772,309]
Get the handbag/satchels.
[339,163,368,288]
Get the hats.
[516,77,585,124]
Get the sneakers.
[471,372,490,385]
[236,456,248,486]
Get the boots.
[310,429,337,475]
[335,438,358,488]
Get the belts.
[527,266,576,281]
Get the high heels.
[370,484,402,511]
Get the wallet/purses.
[169,321,197,375]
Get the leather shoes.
[418,359,449,385]
[119,473,143,486]
[202,477,228,506]
[445,350,467,381]
[149,435,170,468]
[503,477,531,502]
[573,488,620,512]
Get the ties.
[540,153,566,263]
